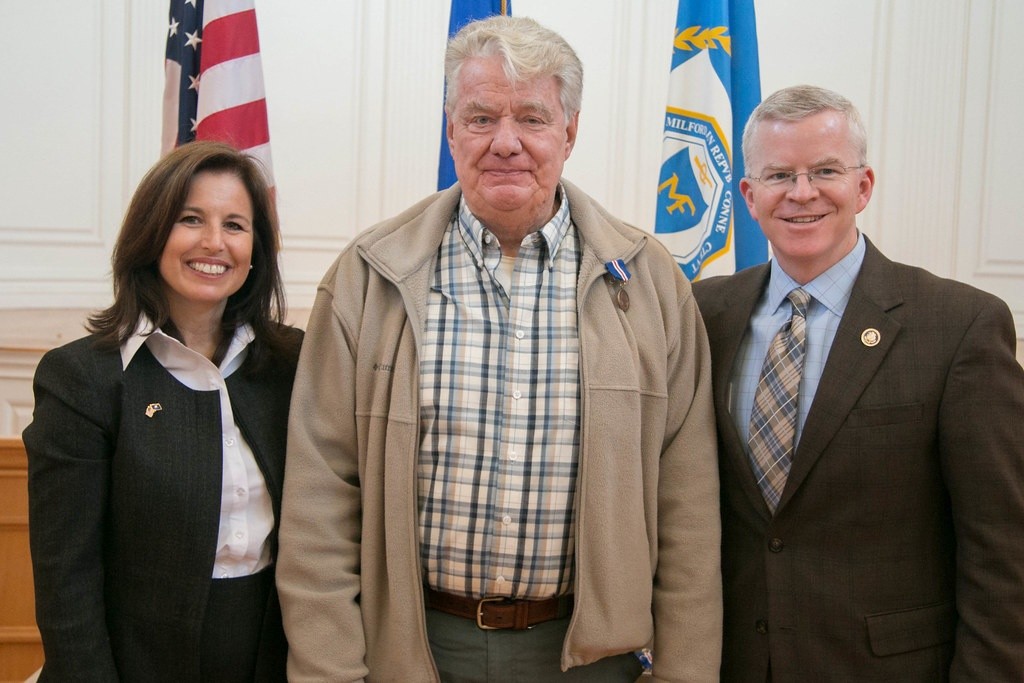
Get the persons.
[275,15,723,683]
[691,85,1024,683]
[22,142,304,683]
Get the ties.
[744,288,812,520]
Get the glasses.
[749,165,868,193]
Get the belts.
[421,587,574,631]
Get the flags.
[162,0,273,196]
[654,0,769,283]
[437,0,511,192]
